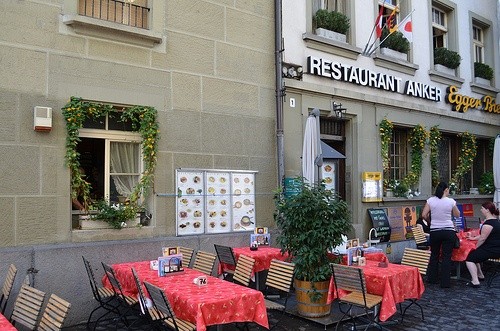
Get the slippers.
[464,277,485,288]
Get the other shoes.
[426,279,440,284]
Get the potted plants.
[380,29,410,61]
[315,9,351,43]
[474,62,493,85]
[434,47,461,76]
[79,201,140,229]
[385,181,396,197]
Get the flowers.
[379,115,478,197]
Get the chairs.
[0,224,430,331]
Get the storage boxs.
[463,216,480,229]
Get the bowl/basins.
[250,247,258,251]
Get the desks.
[102,230,480,331]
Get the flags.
[376,7,382,38]
[398,15,413,43]
[388,8,398,32]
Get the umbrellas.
[493,135,500,209]
[302,108,323,189]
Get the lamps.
[333,102,347,121]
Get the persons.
[421,182,460,287]
[417,207,430,246]
[466,201,500,287]
